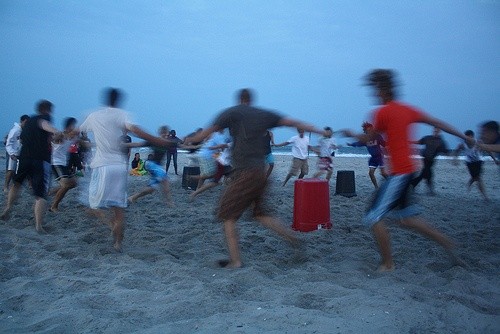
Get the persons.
[264,127,277,180]
[3,114,234,214]
[469,120,500,164]
[179,87,334,268]
[453,131,499,202]
[334,69,475,275]
[312,127,342,187]
[270,127,320,189]
[1,99,68,235]
[347,122,391,194]
[63,87,180,252]
[407,124,457,196]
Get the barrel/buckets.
[333,170,357,198]
[181,166,201,191]
[291,179,332,232]
